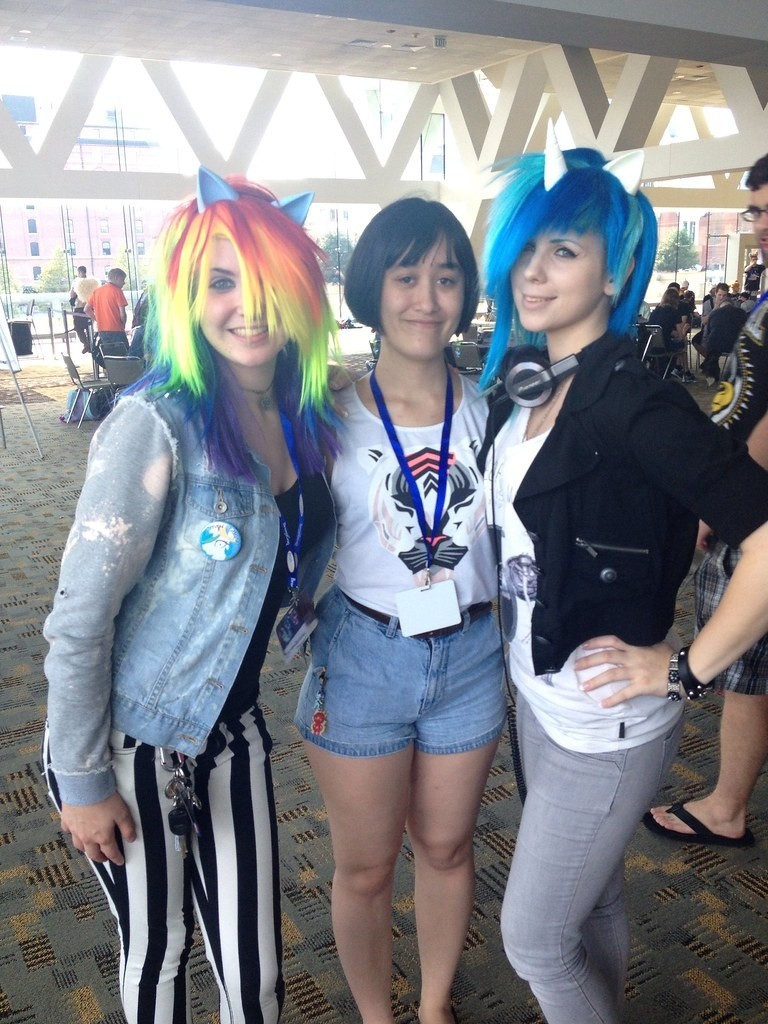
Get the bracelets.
[667,645,715,703]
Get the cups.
[682,316,687,322]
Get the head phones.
[498,329,621,407]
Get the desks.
[447,337,516,368]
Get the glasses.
[740,203,768,222]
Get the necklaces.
[522,375,562,440]
[237,381,275,410]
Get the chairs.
[687,313,694,369]
[99,342,128,392]
[369,339,381,360]
[632,323,687,384]
[451,340,483,374]
[463,325,479,340]
[103,356,145,394]
[60,351,114,429]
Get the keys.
[159,746,202,859]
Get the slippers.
[642,794,759,849]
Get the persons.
[42,175,348,1024]
[293,151,768,1024]
